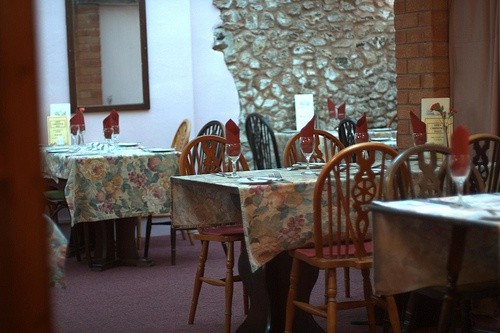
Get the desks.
[363,192,500,333]
[170,159,443,333]
[42,146,181,271]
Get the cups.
[354,131,367,144]
[412,132,426,146]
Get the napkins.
[103,110,119,139]
[226,118,240,157]
[449,122,469,177]
[355,113,368,144]
[300,115,315,153]
[328,99,347,119]
[70,110,85,135]
[410,110,427,145]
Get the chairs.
[137,113,500,333]
[44,188,81,267]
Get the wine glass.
[299,136,316,174]
[225,143,242,178]
[328,111,345,132]
[69,124,119,152]
[448,153,471,206]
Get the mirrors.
[65,0,150,114]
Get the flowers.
[430,102,458,148]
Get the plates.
[151,148,176,152]
[350,169,386,174]
[118,143,138,147]
[293,163,325,168]
[239,178,280,184]
[46,149,69,153]
